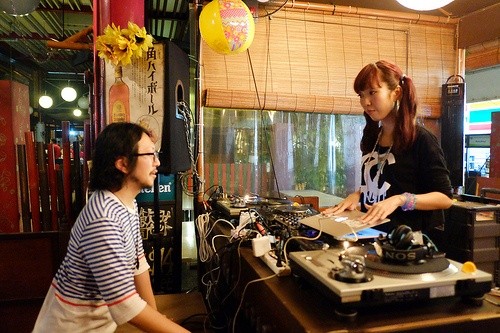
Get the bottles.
[109,65,131,124]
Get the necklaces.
[112,191,140,270]
[365,129,396,169]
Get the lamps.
[78,94,89,109]
[60,80,77,102]
[38,90,53,109]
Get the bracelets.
[403,192,416,211]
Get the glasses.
[136,152,159,162]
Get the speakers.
[150,40,190,172]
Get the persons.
[322,60,454,245]
[50,138,61,173]
[32,123,190,333]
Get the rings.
[335,205,338,207]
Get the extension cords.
[231,228,260,240]
[260,251,291,277]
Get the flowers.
[94,20,155,70]
